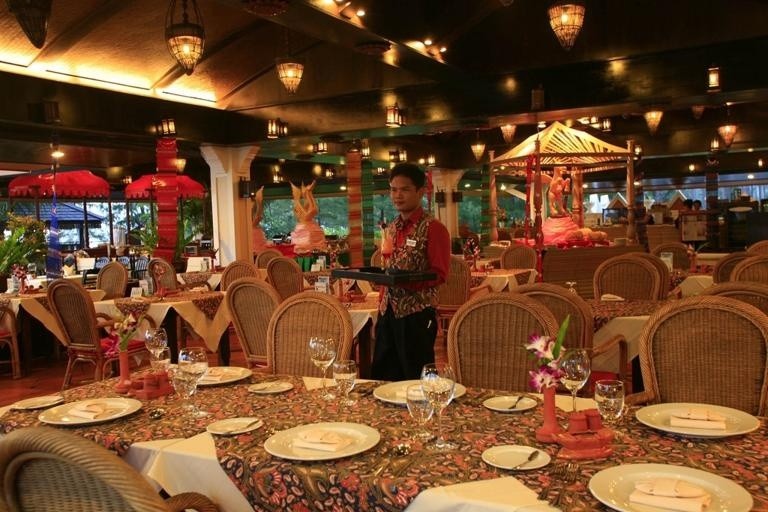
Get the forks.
[538,461,576,507]
[358,381,378,397]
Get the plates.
[207,418,263,435]
[636,403,760,439]
[196,366,252,386]
[264,422,380,461]
[11,396,142,426]
[249,383,293,394]
[588,465,753,512]
[481,445,551,470]
[373,380,467,406]
[483,396,538,412]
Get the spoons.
[363,443,423,478]
[512,450,539,470]
[509,395,525,409]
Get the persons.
[675,199,693,227]
[693,200,701,210]
[371,163,450,380]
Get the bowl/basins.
[614,238,628,246]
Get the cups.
[595,379,625,425]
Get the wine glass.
[420,363,459,451]
[382,239,394,276]
[406,384,436,441]
[308,336,336,400]
[557,349,591,428]
[333,361,358,407]
[145,329,209,418]
[565,281,578,297]
